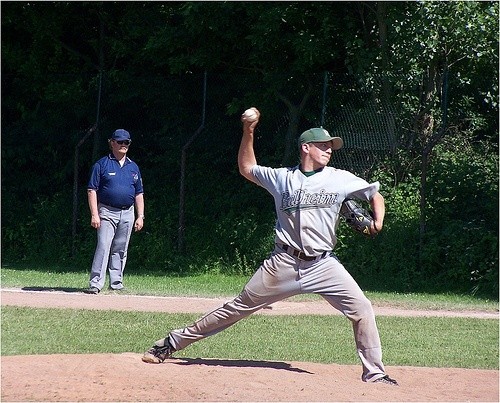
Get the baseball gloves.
[339,198,378,236]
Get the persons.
[142,106,401,386]
[86,127,145,293]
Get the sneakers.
[88,287,98,294]
[372,375,399,386]
[142,337,176,364]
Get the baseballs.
[244,108,257,123]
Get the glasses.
[113,140,129,145]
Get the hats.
[111,129,132,142]
[298,128,343,152]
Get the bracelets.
[374,221,379,233]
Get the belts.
[283,242,332,261]
[100,201,133,210]
